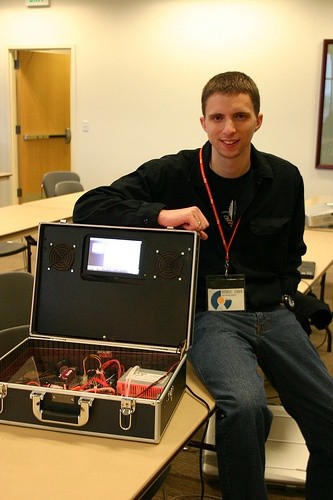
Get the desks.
[0,197,333,500]
[0,191,88,242]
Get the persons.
[73,72,333,500]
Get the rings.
[198,222,200,225]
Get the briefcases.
[0,220,201,444]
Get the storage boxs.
[0,220,201,445]
[202,404,309,490]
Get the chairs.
[41,171,84,199]
[0,234,37,274]
